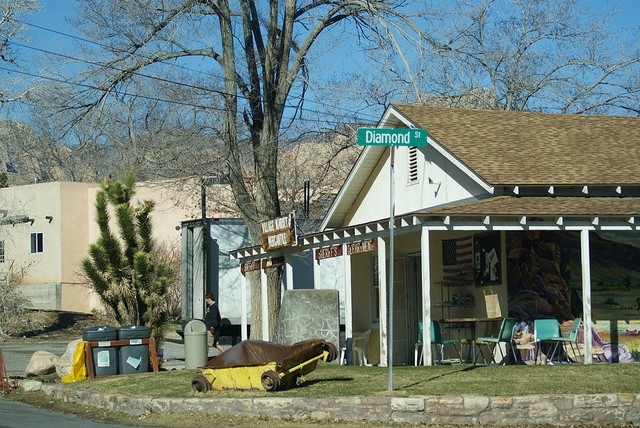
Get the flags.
[441,236,475,280]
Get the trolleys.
[192,338,338,392]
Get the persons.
[205,292,225,352]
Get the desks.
[444,317,505,360]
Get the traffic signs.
[357,127,428,147]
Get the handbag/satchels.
[483,288,502,318]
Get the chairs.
[474,317,518,366]
[340,330,372,366]
[533,319,562,365]
[417,320,463,366]
[545,318,583,364]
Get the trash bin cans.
[184,320,208,370]
[117,325,150,374]
[82,326,118,376]
